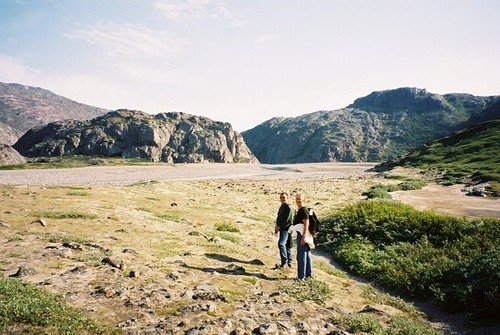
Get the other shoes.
[283,263,292,268]
[276,264,287,269]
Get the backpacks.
[308,208,321,237]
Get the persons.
[274,192,294,269]
[287,193,316,282]
